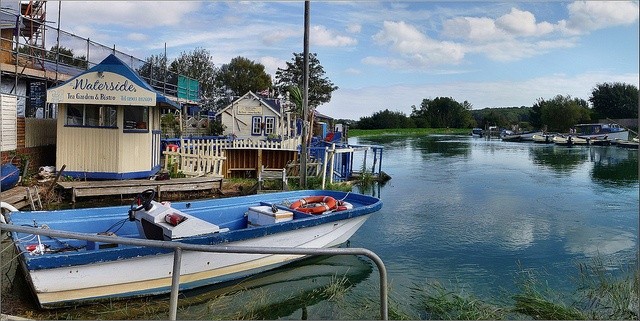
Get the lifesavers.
[289,196,336,214]
[336,200,353,210]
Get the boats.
[532,133,555,144]
[8,189,383,309]
[554,123,629,146]
[500,128,532,141]
[616,133,639,146]
